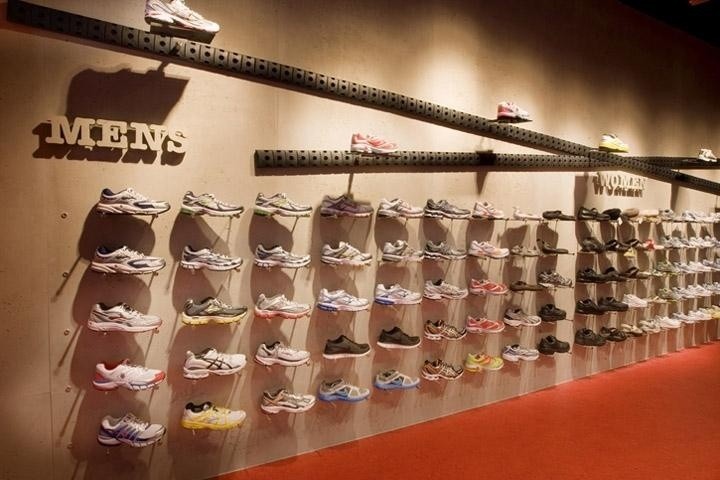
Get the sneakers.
[640,318,659,336]
[180,294,250,327]
[673,310,695,326]
[688,305,719,322]
[461,350,506,374]
[90,243,168,277]
[85,300,163,334]
[464,309,504,336]
[180,243,244,273]
[321,239,375,268]
[259,385,318,417]
[178,189,251,220]
[496,98,530,121]
[373,280,424,306]
[467,277,512,298]
[316,285,373,314]
[575,298,608,317]
[655,281,719,300]
[180,345,249,381]
[423,238,469,261]
[91,356,168,392]
[509,278,547,292]
[619,264,638,277]
[536,333,572,355]
[626,232,638,247]
[180,398,248,432]
[253,292,312,319]
[515,204,540,221]
[255,191,314,220]
[423,278,470,302]
[322,333,373,361]
[95,185,170,216]
[253,240,312,269]
[466,238,510,259]
[511,239,540,257]
[251,338,312,368]
[97,412,166,449]
[377,324,423,350]
[350,131,399,154]
[621,204,640,222]
[580,232,602,252]
[598,295,630,312]
[540,269,575,288]
[602,207,621,223]
[600,324,627,343]
[544,209,576,222]
[666,234,719,249]
[502,342,542,363]
[502,305,541,330]
[542,238,571,255]
[603,236,629,252]
[660,203,679,217]
[536,302,566,322]
[574,328,606,346]
[576,264,610,284]
[635,267,650,279]
[376,189,427,220]
[579,206,612,222]
[380,238,423,262]
[422,319,470,342]
[604,267,630,282]
[597,131,627,149]
[143,0,222,35]
[641,235,663,250]
[420,356,464,384]
[697,145,716,163]
[372,370,423,391]
[423,197,468,218]
[683,207,719,222]
[622,322,643,337]
[317,377,371,402]
[641,206,661,219]
[621,293,648,309]
[652,313,679,331]
[319,188,375,219]
[470,197,508,219]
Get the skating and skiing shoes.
[671,261,696,275]
[656,258,676,276]
[689,261,711,274]
[703,257,720,271]
